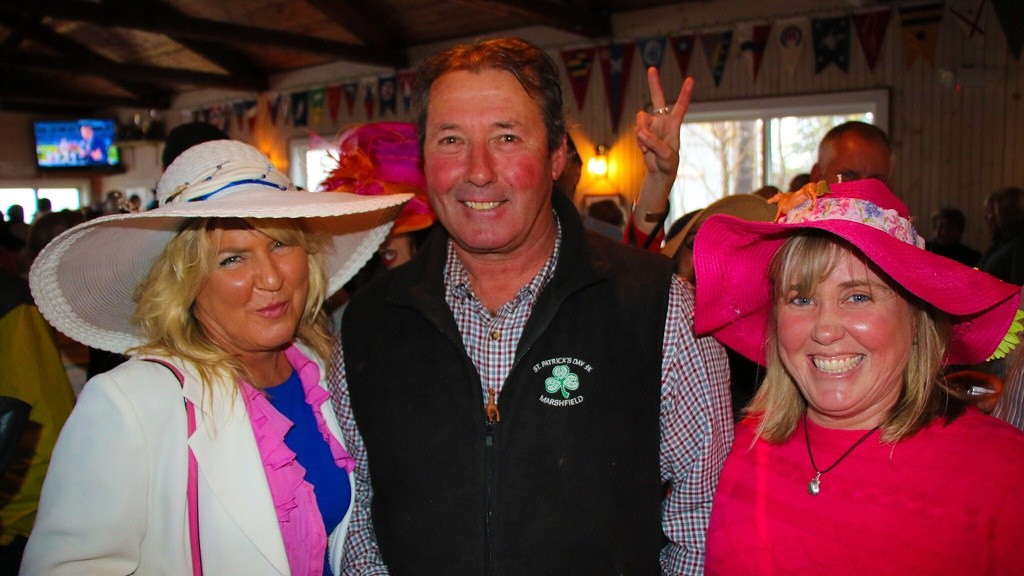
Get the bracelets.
[630,199,669,222]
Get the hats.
[319,120,436,235]
[29,140,413,357]
[692,179,1021,369]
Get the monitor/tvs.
[31,117,124,172]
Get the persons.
[19,140,412,575]
[0,122,225,575]
[75,125,108,166]
[329,37,732,576]
[624,66,775,286]
[808,122,890,182]
[692,179,1024,575]
[325,121,439,272]
[926,185,1024,431]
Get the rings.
[654,107,668,114]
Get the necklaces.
[804,409,883,495]
